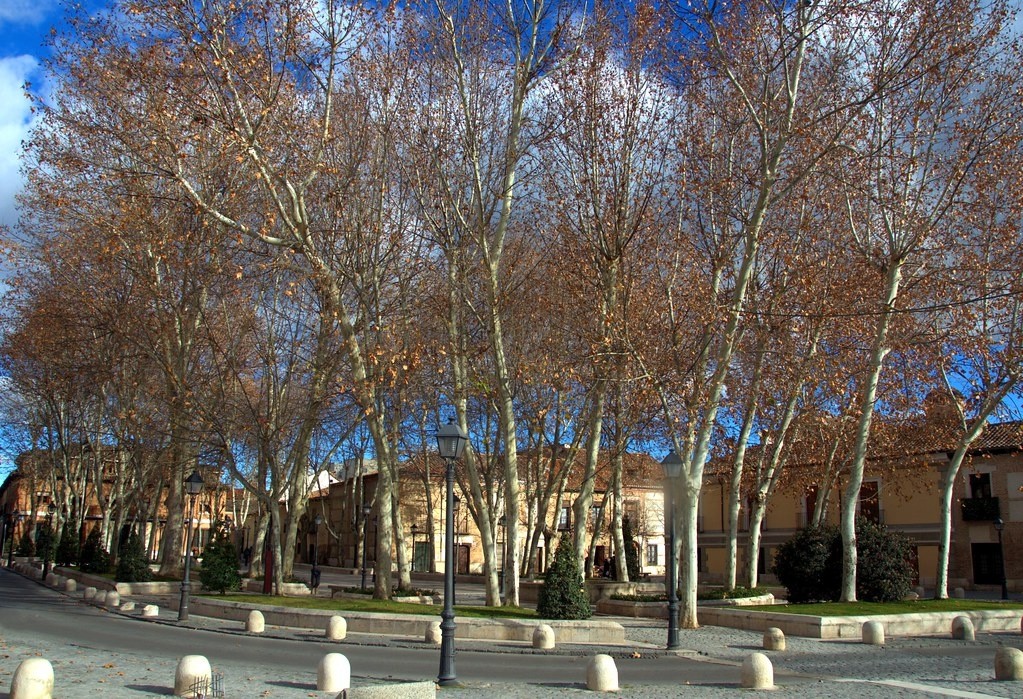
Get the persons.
[603,554,610,577]
[610,556,617,581]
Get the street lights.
[178,470,205,622]
[7,509,19,567]
[410,524,418,572]
[434,418,465,687]
[358,501,371,589]
[42,501,58,580]
[312,513,322,588]
[994,518,1009,600]
[660,448,684,650]
[372,515,378,568]
[444,492,461,605]
